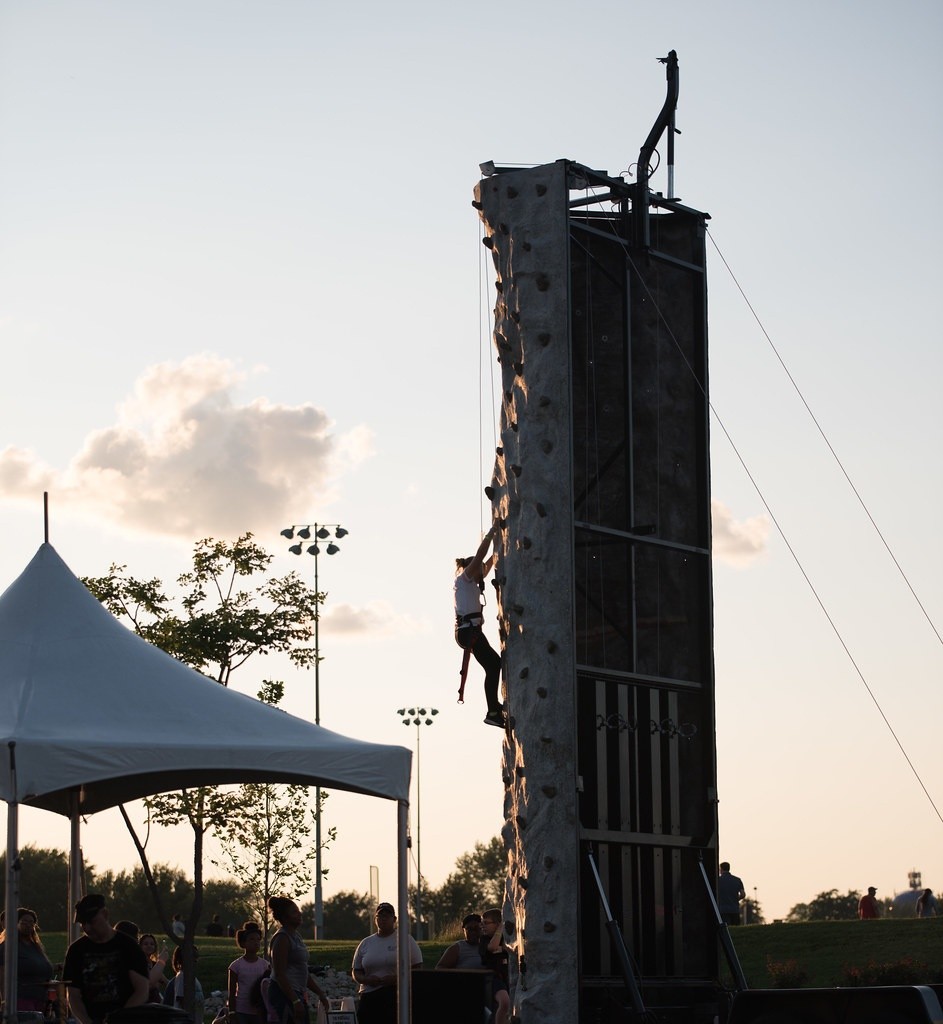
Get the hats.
[375,903,395,916]
[74,894,106,923]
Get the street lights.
[396,708,439,941]
[280,523,349,941]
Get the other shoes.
[483,713,507,729]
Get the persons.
[226,922,271,1024]
[857,887,881,920]
[0,908,62,1012]
[434,907,511,1024]
[268,897,329,1024]
[717,862,746,926]
[456,518,507,728]
[207,914,225,937]
[915,889,939,918]
[62,894,205,1024]
[351,903,423,1024]
[172,913,185,936]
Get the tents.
[0,545,412,1024]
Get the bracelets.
[486,534,493,542]
[495,928,502,936]
[292,999,301,1006]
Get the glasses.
[479,921,497,928]
